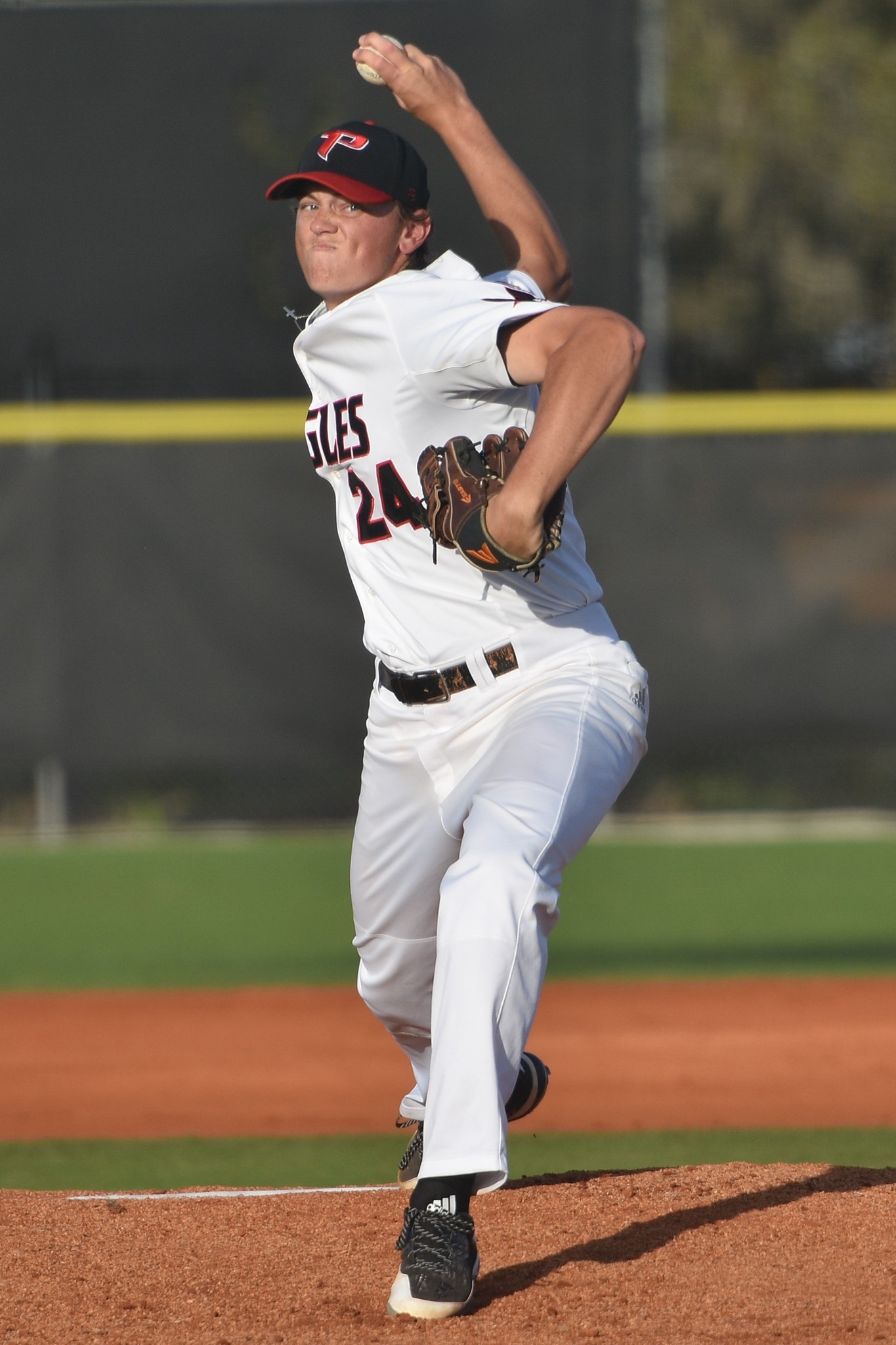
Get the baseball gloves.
[417,427,568,573]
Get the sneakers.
[395,1053,548,1189]
[389,1190,480,1320]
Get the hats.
[268,121,429,209]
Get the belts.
[377,644,518,706]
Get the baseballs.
[355,35,408,85]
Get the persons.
[266,31,650,1320]
